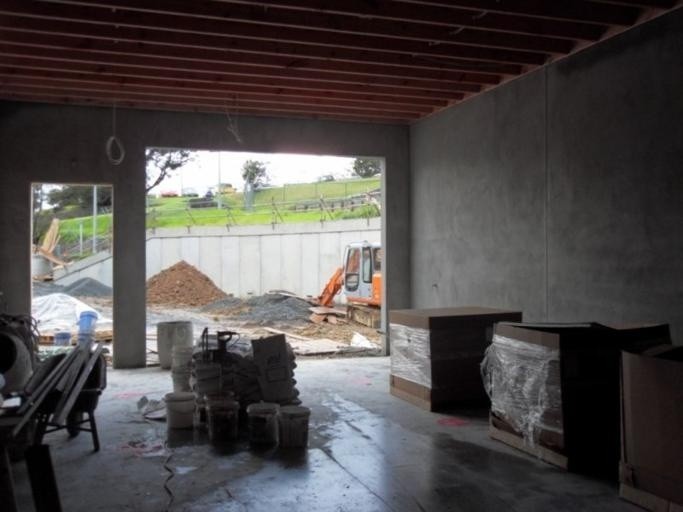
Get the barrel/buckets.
[205,400,242,444]
[156,320,194,370]
[161,390,198,431]
[53,331,72,347]
[245,402,280,451]
[170,369,192,392]
[276,403,313,450]
[169,343,193,374]
[76,332,96,349]
[78,310,99,332]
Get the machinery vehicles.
[306,238,382,329]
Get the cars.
[159,182,237,197]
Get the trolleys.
[24,352,107,454]
